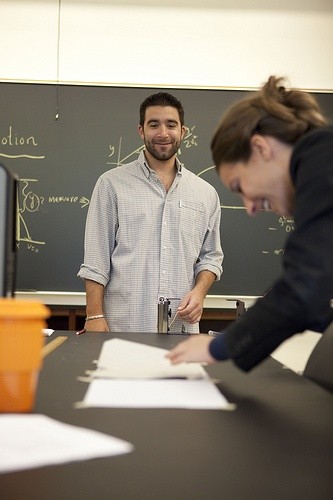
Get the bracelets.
[85,313,105,322]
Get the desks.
[0,321,332,500]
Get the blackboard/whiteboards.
[2,79,332,308]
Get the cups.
[0,297,51,412]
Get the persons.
[76,91,225,336]
[159,74,333,375]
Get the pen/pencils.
[74,328,88,336]
